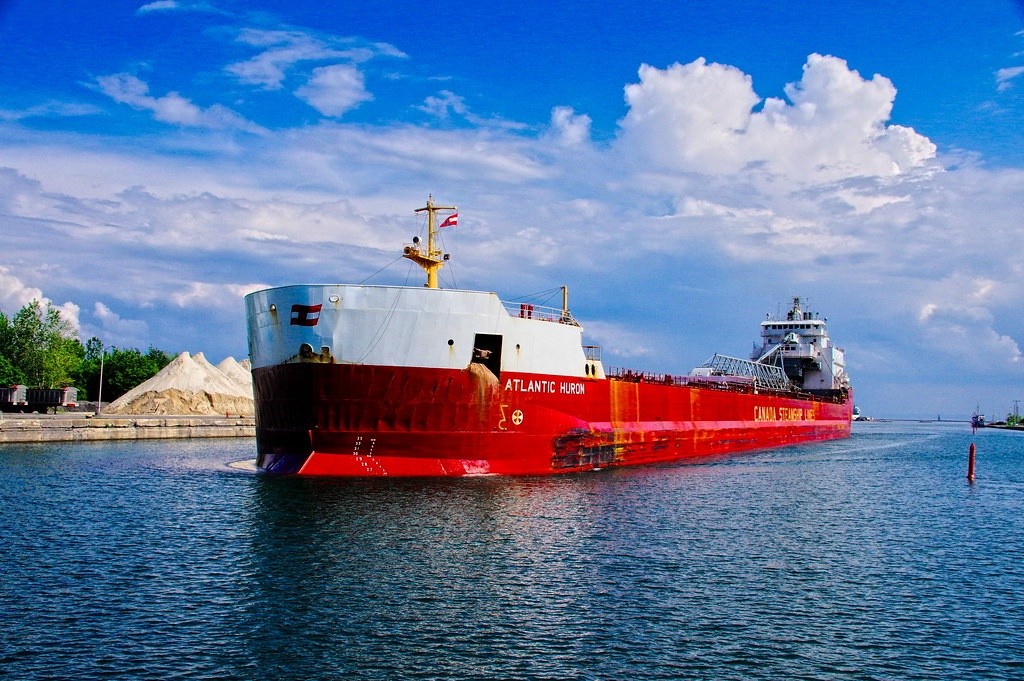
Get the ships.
[243,195,857,477]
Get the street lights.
[97,344,117,411]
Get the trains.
[0,385,80,413]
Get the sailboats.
[971,403,985,428]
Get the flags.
[440,213,457,227]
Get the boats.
[920,420,932,423]
[856,416,874,421]
[852,405,860,421]
